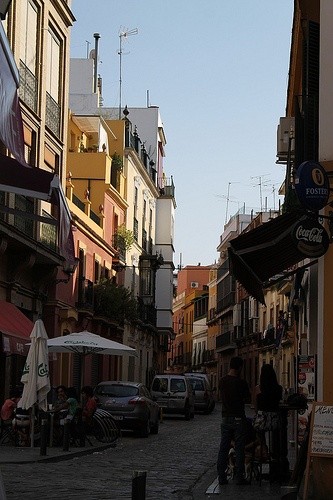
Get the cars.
[93,381,162,438]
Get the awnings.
[0,153,72,225]
[227,206,320,307]
[0,300,58,361]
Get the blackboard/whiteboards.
[309,402,333,457]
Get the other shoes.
[219,473,229,485]
[236,471,248,485]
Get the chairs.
[0,405,95,448]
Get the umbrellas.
[24,330,137,408]
[16,318,51,447]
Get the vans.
[184,373,217,413]
[151,374,196,418]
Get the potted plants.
[115,234,127,256]
[85,281,138,325]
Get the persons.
[217,356,252,485]
[1,386,97,452]
[253,363,289,460]
